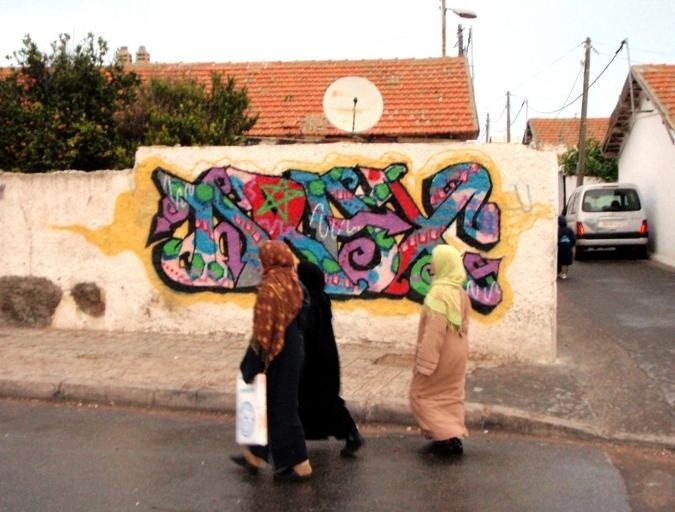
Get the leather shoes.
[230,436,463,485]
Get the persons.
[409,245,471,456]
[558,216,576,279]
[227,239,314,484]
[297,260,363,455]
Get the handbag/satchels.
[234,370,270,446]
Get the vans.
[563,183,648,260]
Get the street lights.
[438,0,478,56]
